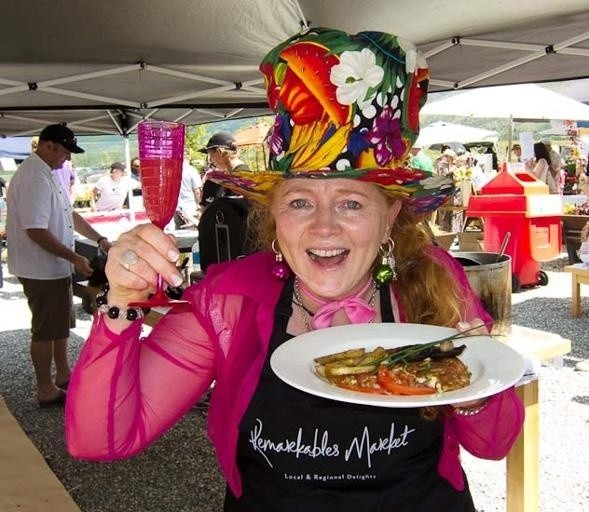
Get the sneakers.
[38,381,70,409]
[192,386,214,416]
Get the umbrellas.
[412,121,501,151]
[417,83,589,162]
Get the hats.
[111,160,126,169]
[512,144,521,150]
[198,131,238,155]
[39,124,86,155]
[205,25,462,227]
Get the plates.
[268,321,528,410]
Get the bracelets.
[95,278,155,323]
[452,402,489,417]
[96,235,108,244]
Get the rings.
[120,249,141,269]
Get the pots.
[450,250,513,339]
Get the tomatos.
[378,366,436,395]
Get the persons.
[4,121,113,408]
[195,131,254,196]
[407,137,568,246]
[64,177,527,512]
[584,152,589,185]
[30,134,80,208]
[88,159,143,212]
[178,151,202,228]
[131,155,143,196]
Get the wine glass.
[126,119,192,308]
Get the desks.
[501,325,577,511]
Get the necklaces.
[293,280,379,333]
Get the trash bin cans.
[465,163,564,291]
